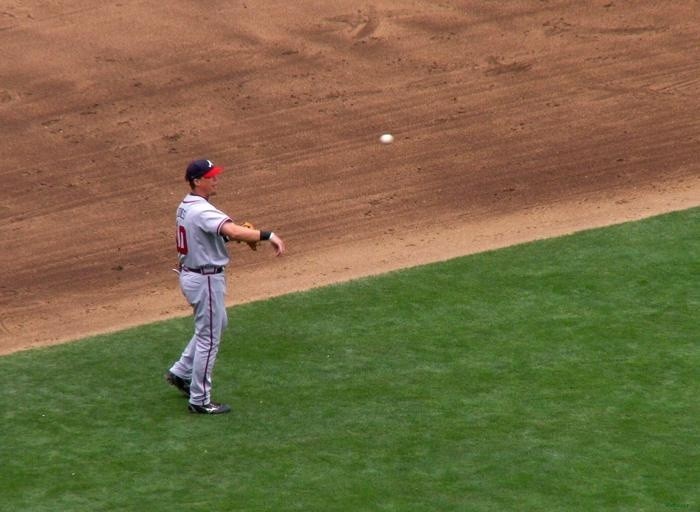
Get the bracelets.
[259,229,273,241]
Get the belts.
[179,265,224,275]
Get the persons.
[162,157,287,416]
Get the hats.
[186,159,222,178]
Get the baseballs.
[379,133,393,143]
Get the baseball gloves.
[236,222,257,252]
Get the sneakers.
[164,370,191,394]
[188,402,229,414]
